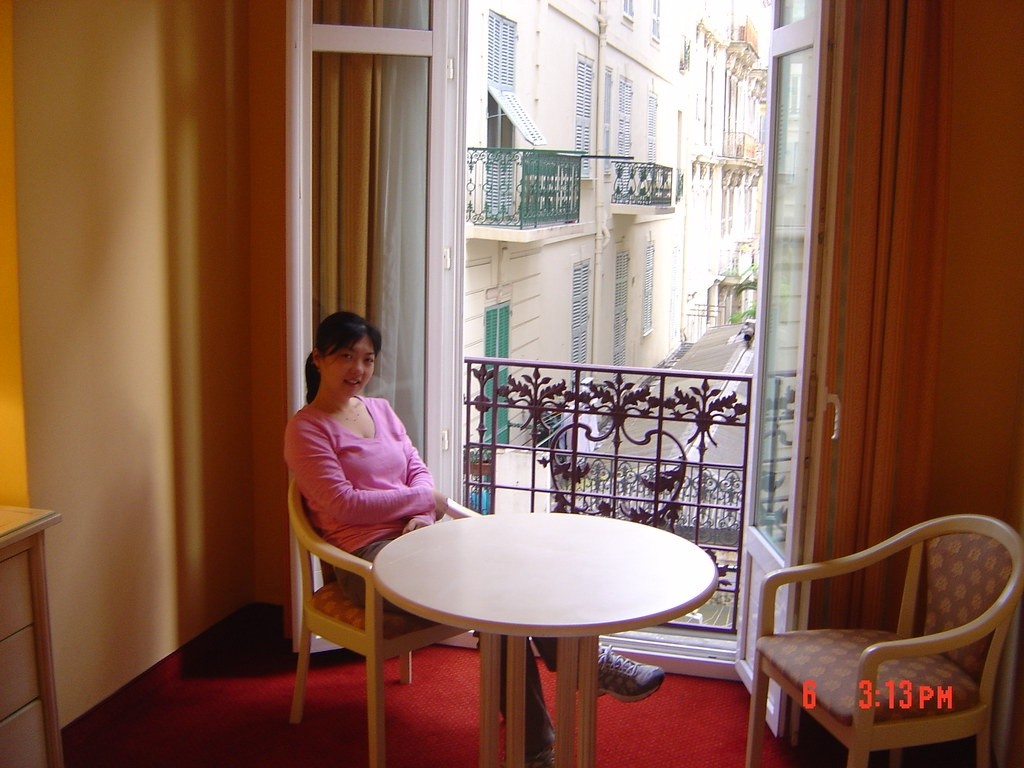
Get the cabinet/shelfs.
[0,501,64,768]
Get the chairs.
[744,514,1024,767]
[288,479,483,768]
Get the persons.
[285,311,666,768]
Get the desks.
[373,513,719,768]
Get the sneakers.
[527,746,556,768]
[595,641,665,702]
